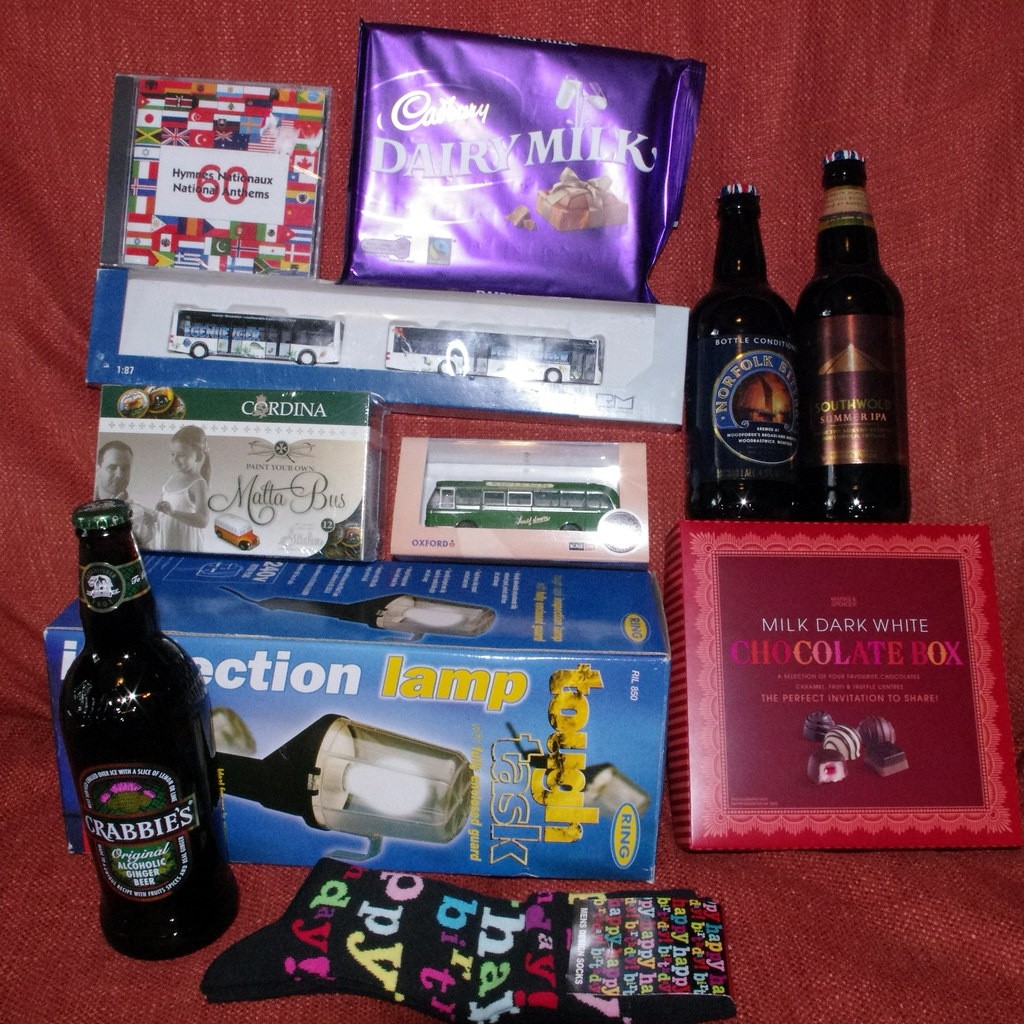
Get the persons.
[156,425,213,552]
[96,441,159,548]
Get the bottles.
[58,499,238,960]
[797,150,910,523]
[684,183,799,522]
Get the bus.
[168,306,345,366]
[424,480,621,530]
[386,325,604,386]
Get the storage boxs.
[95,384,388,562]
[86,266,688,429]
[43,554,671,883]
[388,438,650,570]
[663,521,1024,853]
